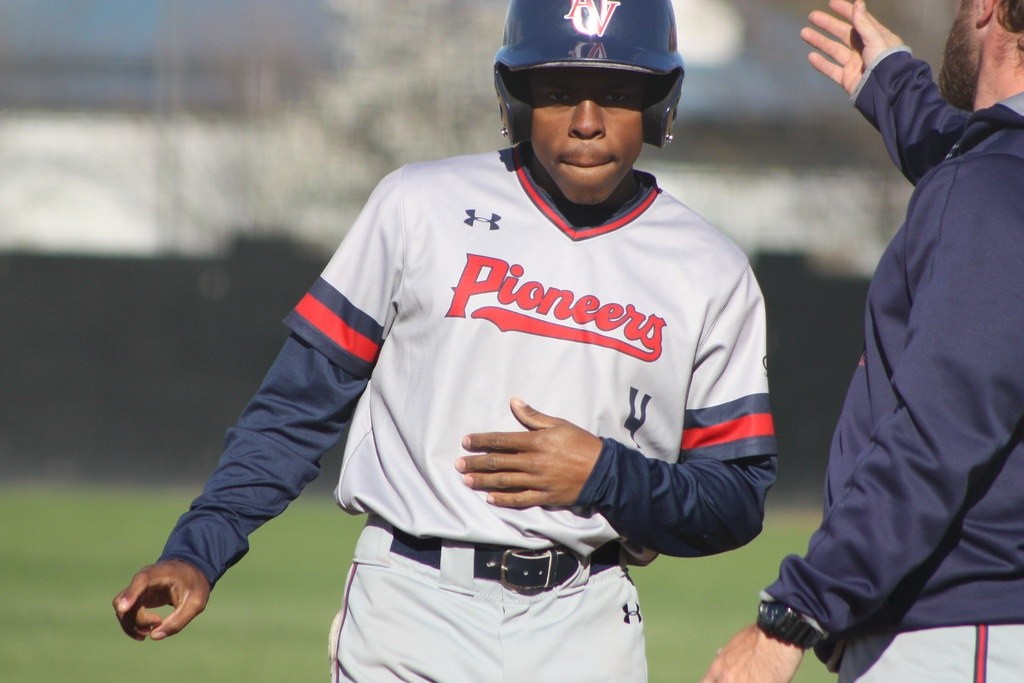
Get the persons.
[112,0,779,683]
[697,0,1024,683]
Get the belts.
[390,527,621,591]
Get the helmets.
[493,0,685,151]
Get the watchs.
[757,600,824,649]
